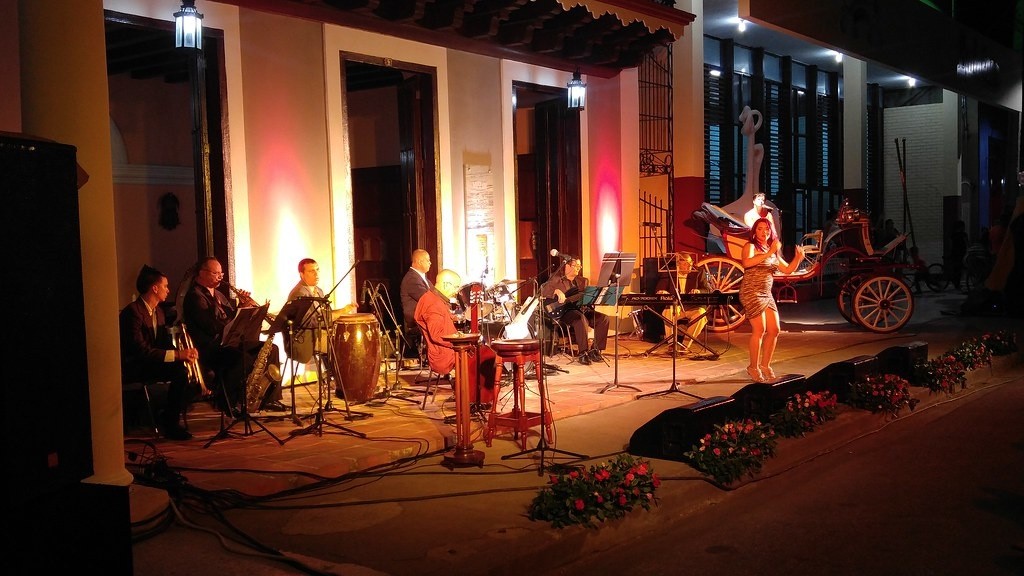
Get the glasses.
[572,263,583,269]
[202,268,224,277]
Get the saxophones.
[243,326,283,416]
[219,278,278,326]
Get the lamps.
[172,0,204,55]
[567,71,587,112]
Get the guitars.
[466,284,497,412]
[540,289,584,321]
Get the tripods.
[203,302,285,450]
[500,293,589,476]
[281,301,442,445]
[636,295,706,400]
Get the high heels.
[745,364,767,383]
[758,363,776,380]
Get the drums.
[486,284,516,314]
[456,281,494,322]
[333,314,382,403]
[480,321,535,377]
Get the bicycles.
[924,256,975,295]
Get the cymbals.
[498,278,527,287]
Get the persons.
[282,258,359,364]
[119,263,215,440]
[401,249,434,339]
[885,219,898,232]
[744,193,778,237]
[184,256,286,419]
[414,269,461,375]
[541,254,609,365]
[655,254,709,351]
[909,246,929,297]
[739,218,805,382]
[949,220,969,289]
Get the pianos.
[618,292,741,358]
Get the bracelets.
[177,350,180,359]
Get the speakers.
[0,131,93,512]
[627,396,735,462]
[874,340,929,383]
[806,355,879,402]
[0,481,134,576]
[730,373,806,425]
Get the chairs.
[543,311,576,358]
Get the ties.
[152,308,157,339]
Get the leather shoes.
[265,399,293,412]
[588,349,604,363]
[579,353,591,365]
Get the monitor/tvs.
[351,165,402,229]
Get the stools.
[487,339,554,452]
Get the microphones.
[772,234,777,258]
[549,249,572,261]
[638,221,662,227]
[359,281,366,305]
[369,284,379,305]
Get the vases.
[739,453,757,474]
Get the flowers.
[769,390,841,439]
[914,327,1018,396]
[527,453,662,530]
[848,372,920,417]
[683,418,778,486]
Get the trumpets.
[178,323,214,402]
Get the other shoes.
[667,349,676,356]
[680,349,688,355]
[159,411,191,440]
[469,402,491,413]
[226,403,248,418]
